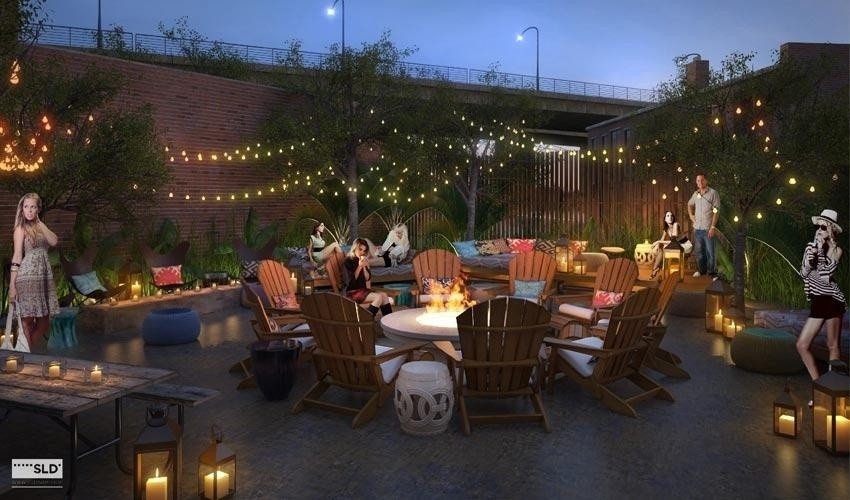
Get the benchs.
[127,382,220,472]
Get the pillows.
[421,276,454,293]
[593,289,624,307]
[150,264,184,285]
[71,270,108,294]
[241,259,260,278]
[571,241,588,255]
[512,279,546,303]
[474,236,510,256]
[272,294,300,309]
[453,239,479,257]
[535,239,556,257]
[506,238,536,253]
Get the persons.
[308,220,341,268]
[687,172,721,278]
[7,192,58,346]
[650,211,692,281]
[796,209,848,407]
[343,237,393,316]
[365,223,410,268]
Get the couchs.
[286,246,422,287]
[452,240,589,284]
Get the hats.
[812,209,843,235]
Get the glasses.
[815,224,827,232]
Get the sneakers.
[693,272,701,277]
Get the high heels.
[649,267,659,280]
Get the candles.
[714,309,723,332]
[827,415,850,452]
[574,264,585,275]
[559,256,567,273]
[48,362,60,377]
[203,466,229,499]
[90,364,101,383]
[146,467,167,500]
[7,359,17,372]
[727,320,742,336]
[778,414,795,435]
[290,272,298,294]
[131,281,141,297]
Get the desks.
[379,307,509,352]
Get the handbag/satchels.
[676,234,693,254]
[1,301,31,353]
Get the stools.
[661,248,685,282]
[245,339,303,402]
[393,360,455,437]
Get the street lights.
[517,26,539,91]
[328,1,345,53]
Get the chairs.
[634,244,655,282]
[541,287,676,418]
[291,290,429,431]
[455,297,552,435]
[58,245,129,305]
[551,257,640,328]
[411,248,471,306]
[228,278,314,392]
[324,251,397,309]
[234,237,277,282]
[638,271,691,379]
[256,260,300,314]
[503,250,557,306]
[143,240,197,290]
